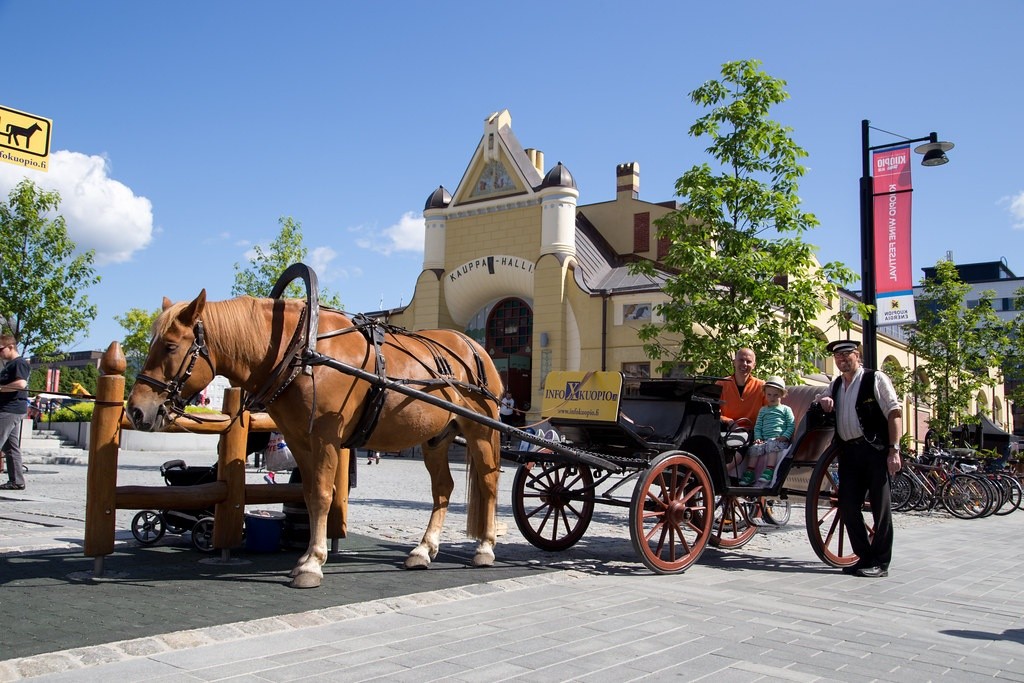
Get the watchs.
[889,444,900,450]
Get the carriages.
[126,262,879,589]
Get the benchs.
[726,386,829,487]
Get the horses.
[126,287,507,589]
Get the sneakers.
[738,471,756,485]
[758,469,773,482]
[0,480,25,490]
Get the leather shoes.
[856,567,888,577]
[843,559,871,574]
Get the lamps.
[540,332,548,347]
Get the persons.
[0,334,31,489]
[817,340,901,577]
[739,375,796,486]
[714,348,769,471]
[366,450,380,465]
[31,396,57,430]
[501,391,520,451]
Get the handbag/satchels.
[265,433,297,471]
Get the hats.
[826,340,861,353]
[761,376,787,397]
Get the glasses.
[833,352,852,358]
[0,344,10,353]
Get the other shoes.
[367,461,372,465]
[376,457,381,464]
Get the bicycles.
[886,426,1023,519]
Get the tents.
[949,412,1019,462]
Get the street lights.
[861,118,954,372]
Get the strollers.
[133,439,223,549]
